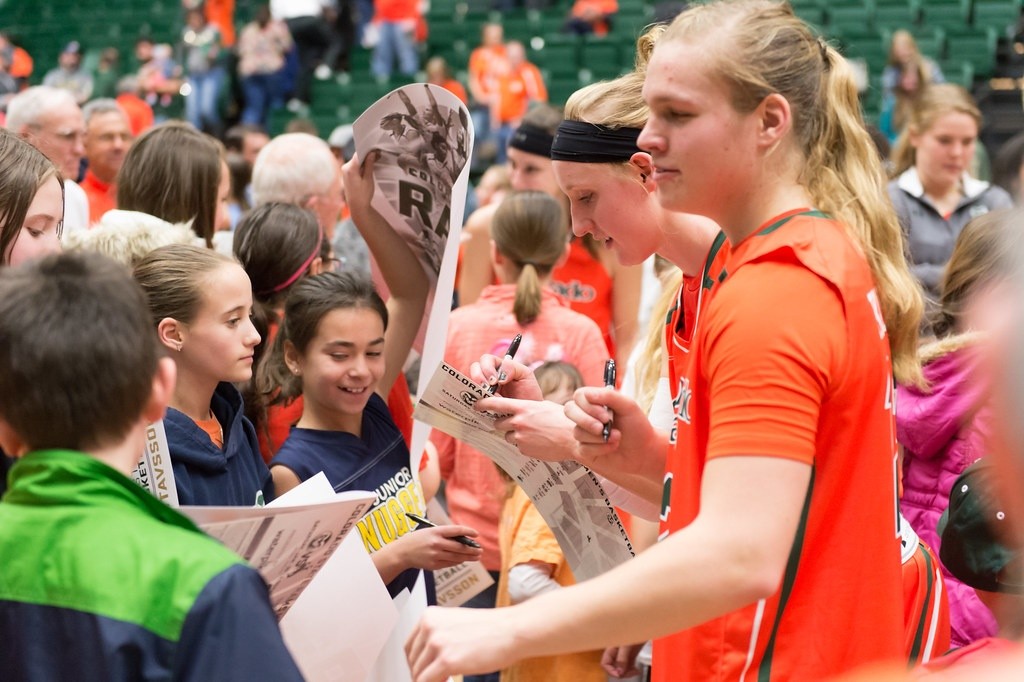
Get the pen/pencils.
[255,490,265,507]
[603,359,617,443]
[487,334,522,394]
[406,512,480,548]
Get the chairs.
[0,0,1024,172]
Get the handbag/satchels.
[939,462,1024,596]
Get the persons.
[45,40,93,104]
[894,207,1024,652]
[561,0,619,36]
[0,31,34,92]
[403,0,952,682]
[888,83,1012,344]
[882,30,946,141]
[133,0,431,133]
[0,86,482,682]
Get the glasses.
[322,257,345,271]
